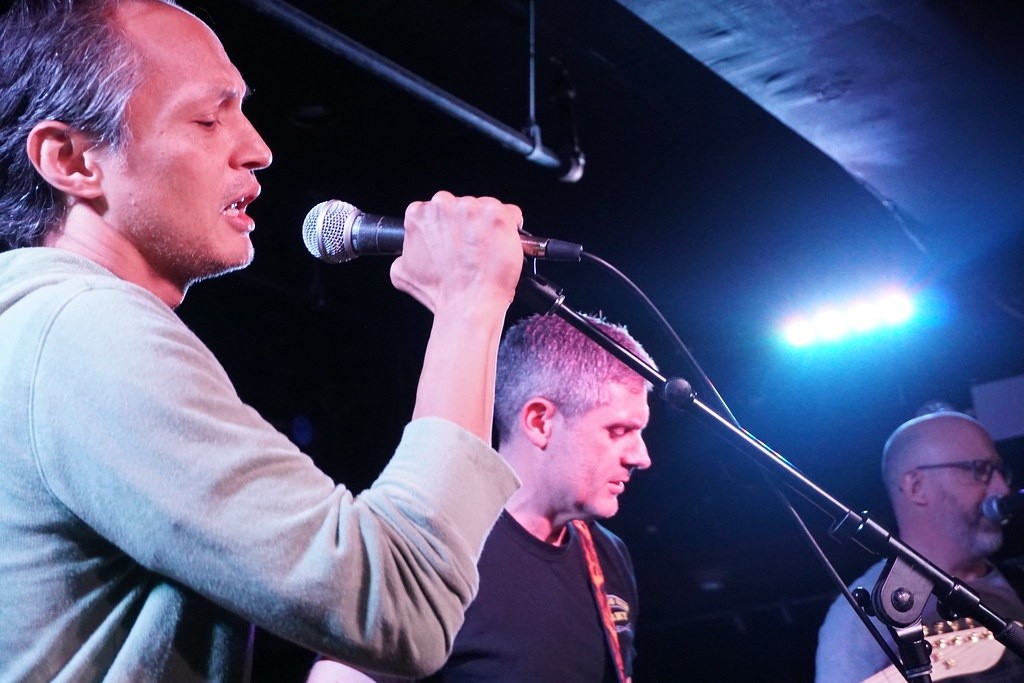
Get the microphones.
[983,489,1024,522]
[302,199,583,264]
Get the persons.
[305,307,659,683]
[0,0,529,683]
[815,410,1024,683]
